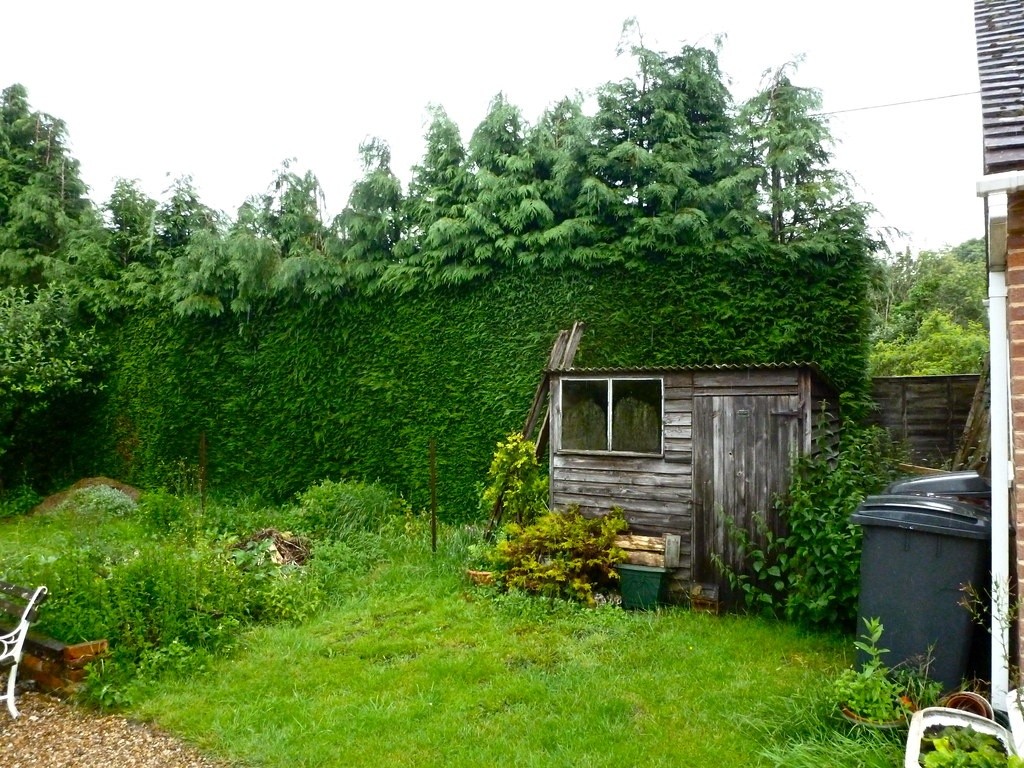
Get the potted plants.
[958,574,1024,760]
[828,616,916,735]
[500,499,632,607]
[464,526,516,585]
[905,706,1024,768]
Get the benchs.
[0,580,50,718]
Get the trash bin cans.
[849,466,992,697]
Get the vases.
[613,565,676,611]
